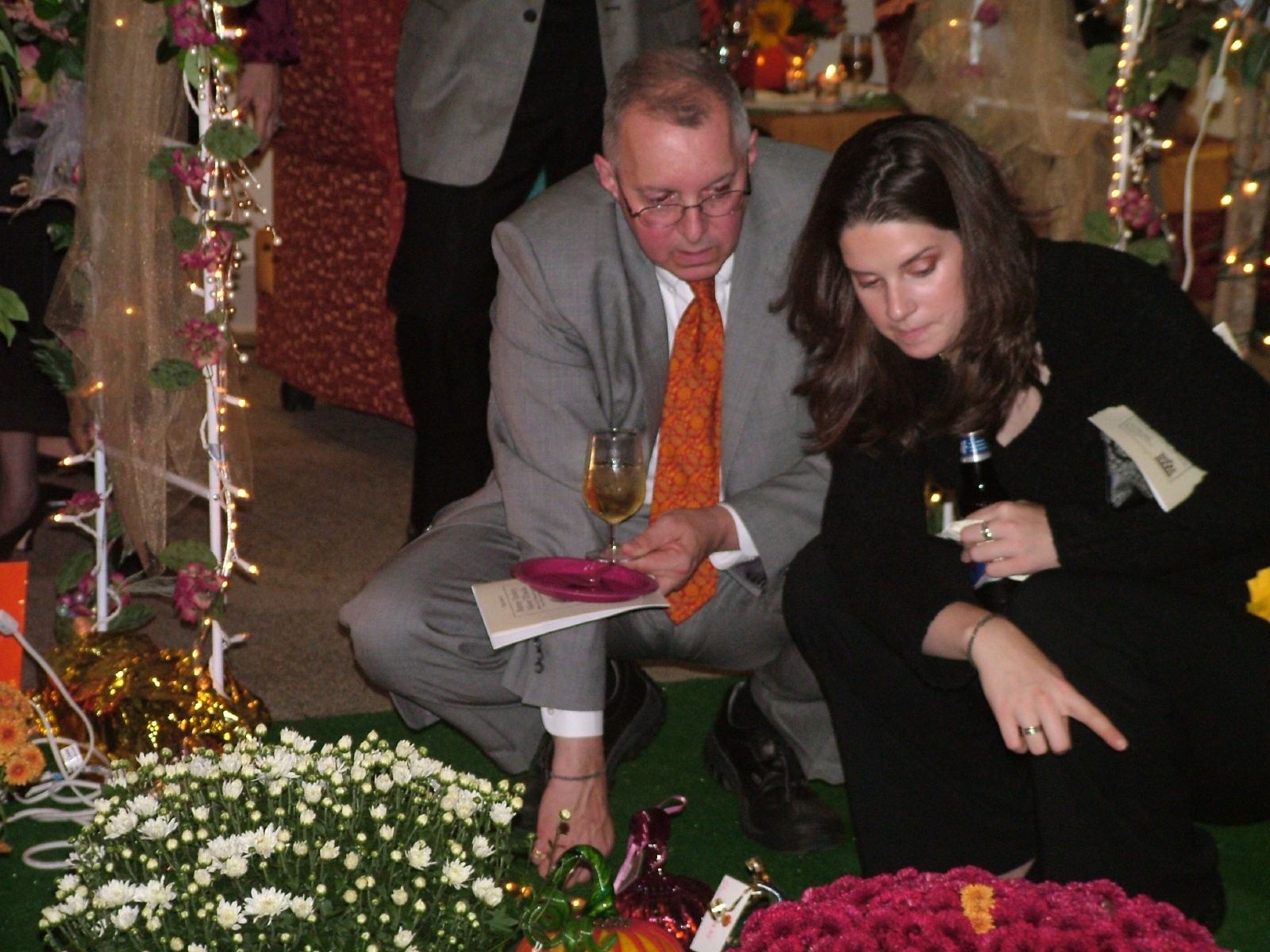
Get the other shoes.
[38,451,95,475]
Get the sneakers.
[505,659,663,853]
[701,681,844,852]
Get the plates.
[511,557,659,603]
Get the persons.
[770,120,1270,934]
[332,43,832,879]
[388,0,703,572]
[0,66,78,548]
[231,0,305,152]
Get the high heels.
[0,499,50,559]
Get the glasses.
[616,148,751,230]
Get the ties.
[649,276,724,625]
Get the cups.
[711,11,753,69]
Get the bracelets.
[548,766,608,781]
[965,611,1002,662]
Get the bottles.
[957,423,1012,583]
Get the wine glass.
[581,427,646,563]
[838,32,874,101]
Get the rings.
[1021,725,1042,734]
[980,521,993,541]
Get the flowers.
[0,0,262,646]
[1083,1,1233,270]
